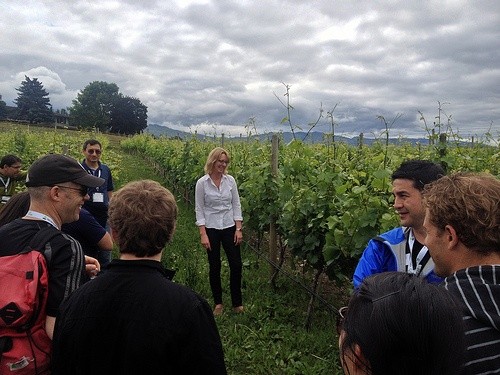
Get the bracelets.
[235,227,244,232]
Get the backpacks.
[0,229,63,375]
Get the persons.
[0,154,105,375]
[52,179,225,374]
[0,155,21,208]
[353,159,448,293]
[338,272,465,375]
[0,191,30,226]
[422,171,500,375]
[79,139,113,260]
[194,147,245,317]
[61,202,112,255]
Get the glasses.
[217,158,229,164]
[87,149,101,154]
[10,166,21,169]
[336,307,349,334]
[49,185,89,196]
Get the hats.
[25,152,105,188]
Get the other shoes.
[213,305,223,316]
[233,305,244,314]
